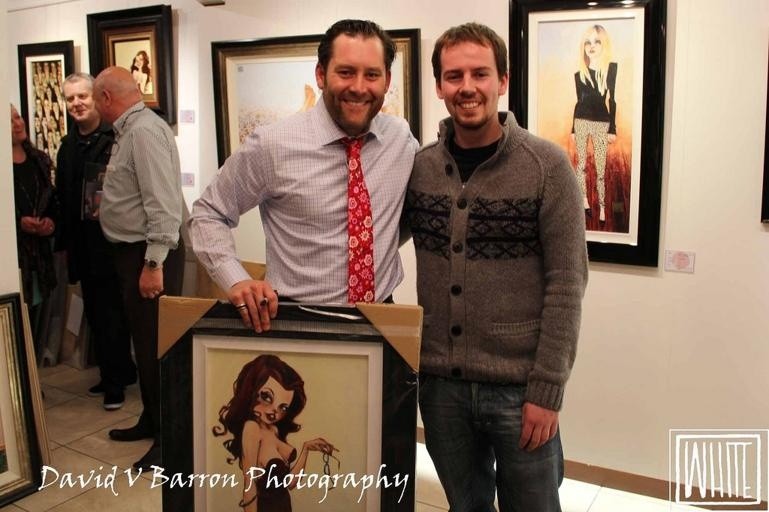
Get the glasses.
[322,451,342,490]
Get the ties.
[338,134,376,304]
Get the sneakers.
[102,382,125,410]
[87,373,138,396]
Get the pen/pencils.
[33,217,40,232]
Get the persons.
[187,17,421,335]
[396,19,592,512]
[132,49,151,93]
[31,61,66,157]
[95,64,184,473]
[210,352,342,512]
[52,73,137,410]
[569,20,620,229]
[11,102,55,401]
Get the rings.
[234,302,247,312]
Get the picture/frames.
[0,292,46,510]
[16,39,74,186]
[211,27,424,176]
[154,293,420,511]
[21,303,53,470]
[507,0,669,269]
[84,2,177,125]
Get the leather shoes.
[584,208,606,228]
[108,417,159,441]
[132,442,162,471]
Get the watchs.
[143,256,161,271]
